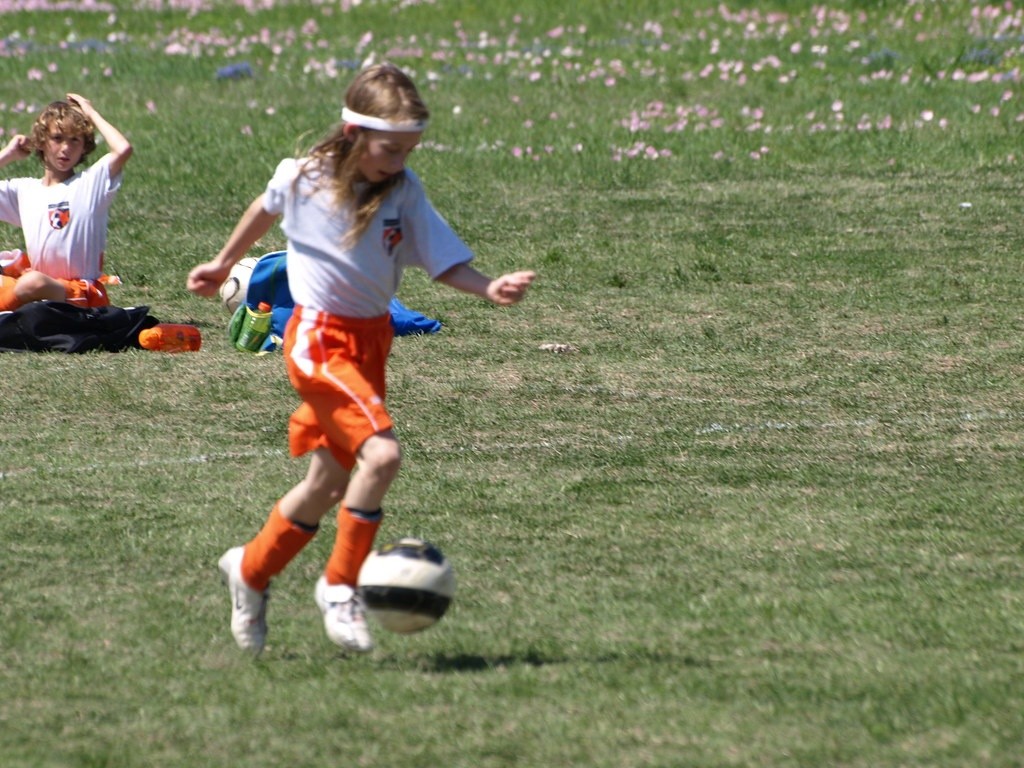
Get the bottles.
[236,302,271,353]
[138,324,201,352]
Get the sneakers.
[217,547,270,659]
[314,575,372,654]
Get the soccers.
[355,536,454,636]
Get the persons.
[0,93,133,314]
[185,65,537,652]
